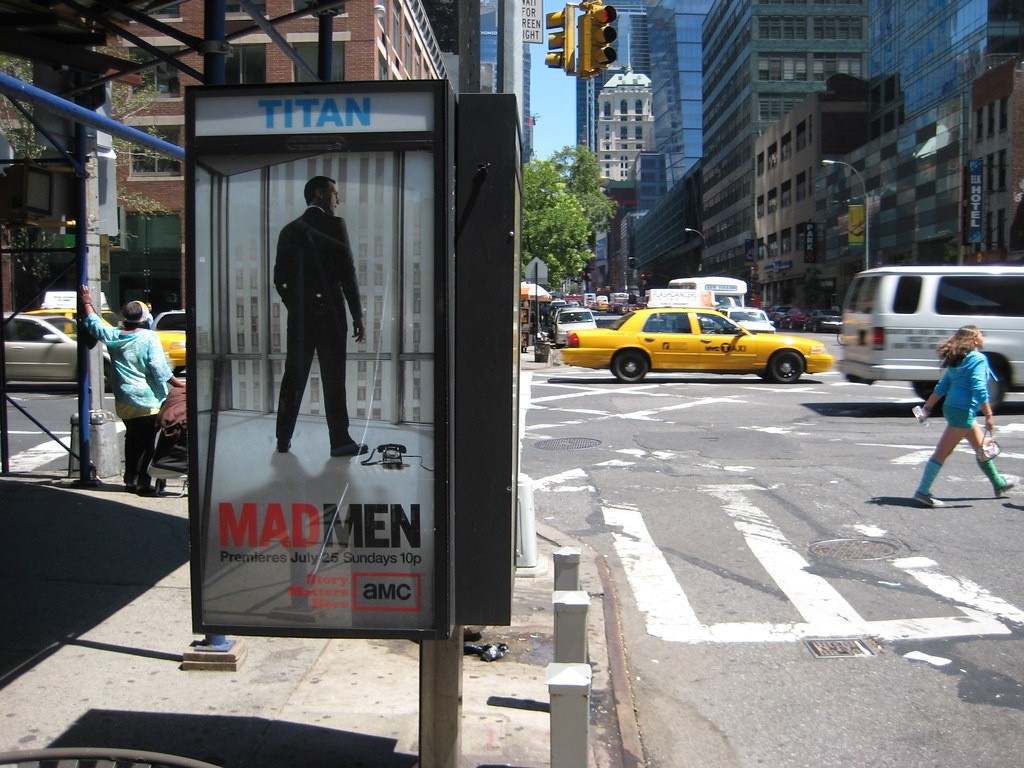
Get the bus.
[666,276,746,305]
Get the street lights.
[628,256,651,290]
[685,228,709,276]
[821,158,871,271]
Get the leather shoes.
[277,440,291,452]
[330,444,368,457]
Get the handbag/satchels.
[978,428,1000,463]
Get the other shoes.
[137,486,155,493]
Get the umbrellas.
[521,281,552,307]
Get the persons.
[911,325,1019,507]
[272,177,368,458]
[78,284,186,495]
[146,303,154,327]
[832,303,840,311]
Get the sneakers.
[994,476,1018,496]
[914,492,943,507]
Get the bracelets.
[924,405,931,412]
[82,302,91,306]
[985,413,992,415]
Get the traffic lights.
[543,6,575,75]
[578,4,617,77]
[0,163,56,224]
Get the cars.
[538,291,843,348]
[152,308,187,375]
[565,308,834,384]
[21,309,127,340]
[3,311,113,390]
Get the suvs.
[834,263,1024,411]
